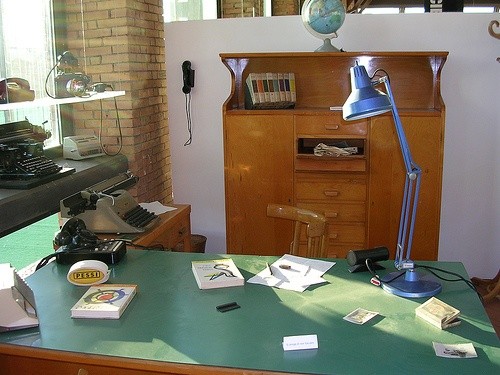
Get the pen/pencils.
[266,261,272,276]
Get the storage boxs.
[415,297,461,329]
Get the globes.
[301,0,346,52]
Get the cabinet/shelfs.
[219,51,449,261]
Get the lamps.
[342,61,441,297]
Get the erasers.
[279,265,291,269]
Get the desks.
[0,204,500,375]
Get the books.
[192,258,244,290]
[70,283,138,320]
[245,71,296,108]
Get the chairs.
[265,204,328,258]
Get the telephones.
[181,61,194,95]
[0,77,35,104]
[53,51,89,97]
[53,216,126,264]
